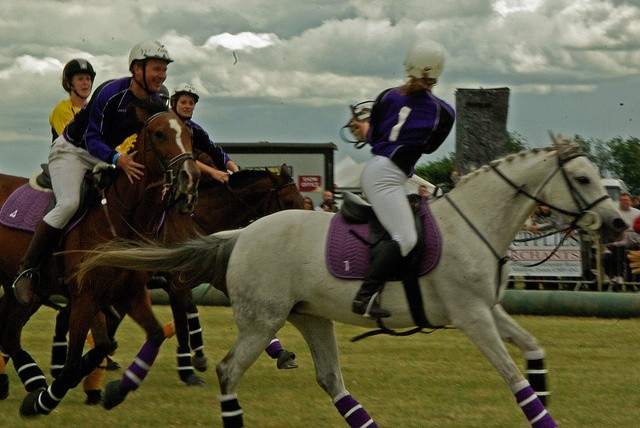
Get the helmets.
[62,59,96,92]
[129,39,174,71]
[404,40,445,80]
[170,83,199,102]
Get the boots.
[11,219,64,309]
[352,240,395,318]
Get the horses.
[55,128,629,428]
[51,162,306,407]
[0,99,201,421]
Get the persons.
[318,191,338,212]
[12,39,173,306]
[352,39,455,316]
[304,195,315,209]
[44,58,97,214]
[618,193,639,231]
[169,82,239,182]
